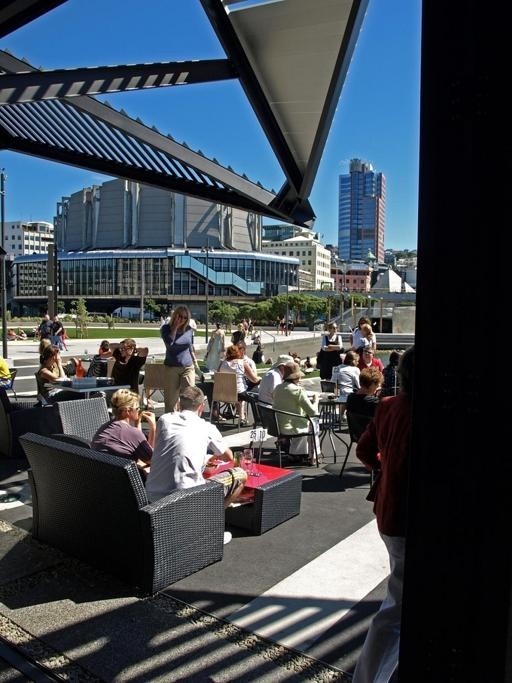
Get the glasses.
[178,314,187,318]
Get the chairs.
[51,397,110,449]
[0,349,145,407]
[141,349,403,482]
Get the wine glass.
[234,449,254,475]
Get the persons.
[350,344,413,683]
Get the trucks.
[112,306,154,321]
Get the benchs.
[19,433,224,596]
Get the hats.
[282,360,305,381]
[268,353,295,369]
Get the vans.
[87,312,107,318]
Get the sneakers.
[223,530,232,544]
[212,412,248,425]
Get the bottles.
[76,357,84,376]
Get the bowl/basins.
[204,465,219,474]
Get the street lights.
[293,266,301,326]
[324,261,386,335]
[200,236,215,343]
[284,264,294,336]
